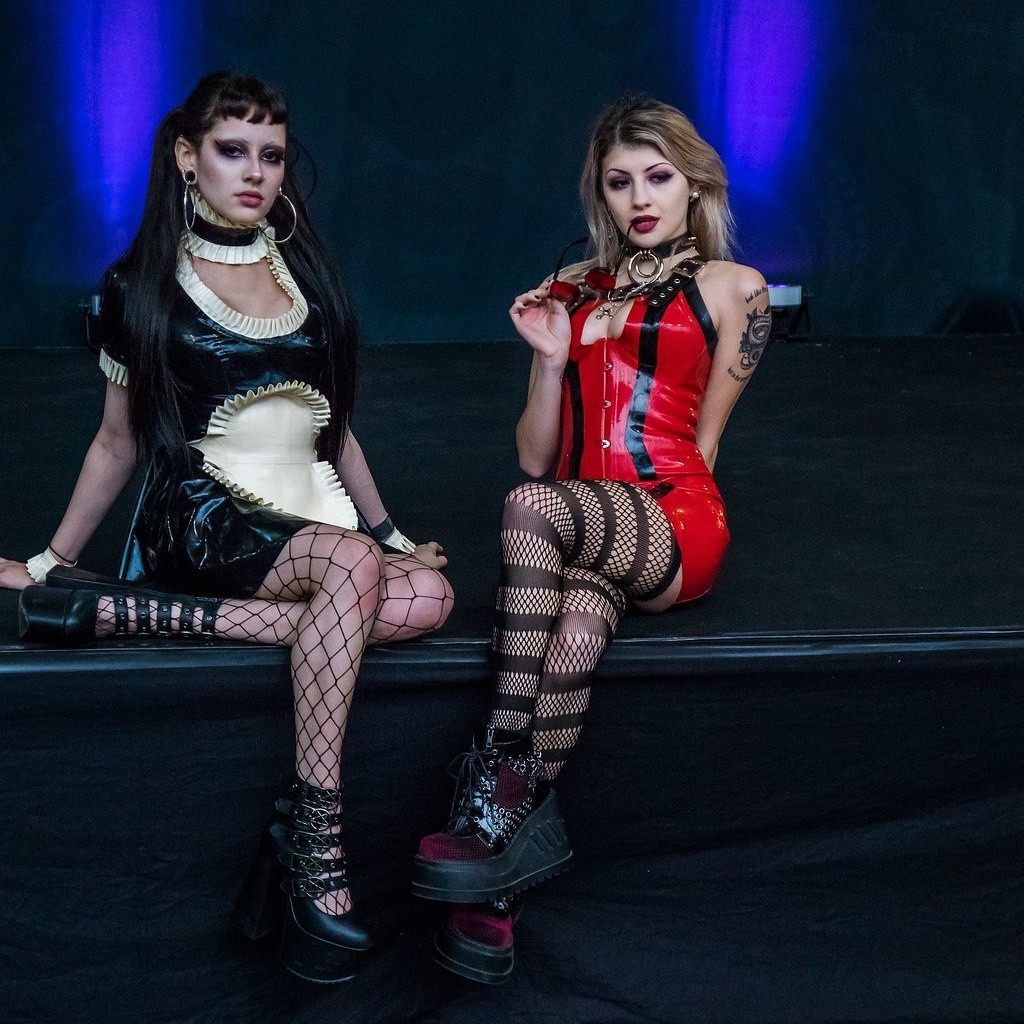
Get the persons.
[1,75,453,984]
[410,100,773,986]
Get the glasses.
[547,215,636,307]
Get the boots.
[229,776,374,949]
[18,559,222,655]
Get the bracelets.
[372,513,394,542]
[48,544,76,564]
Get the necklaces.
[596,231,695,320]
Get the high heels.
[410,743,573,908]
[426,891,518,988]
